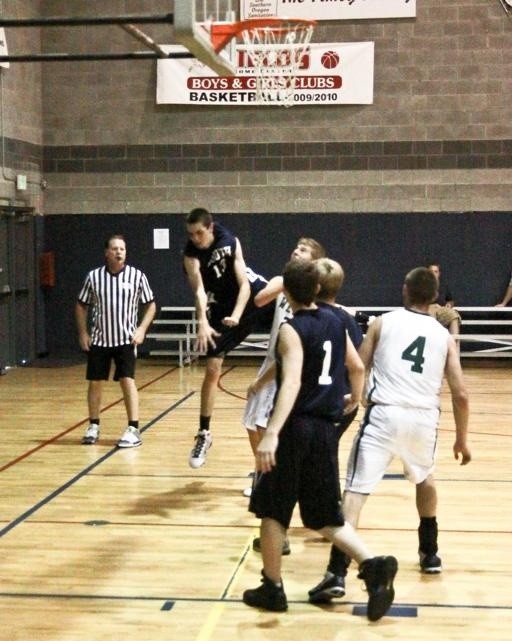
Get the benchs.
[146,302,512,370]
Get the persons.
[181,208,380,470]
[74,234,157,449]
[307,267,471,603]
[428,263,461,360]
[252,257,367,555]
[494,278,511,309]
[240,237,329,501]
[243,258,399,623]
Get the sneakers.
[418,551,442,573]
[253,537,290,555]
[119,426,141,448]
[360,556,397,621]
[244,578,288,611]
[189,429,212,468]
[81,424,99,444]
[309,571,345,601]
